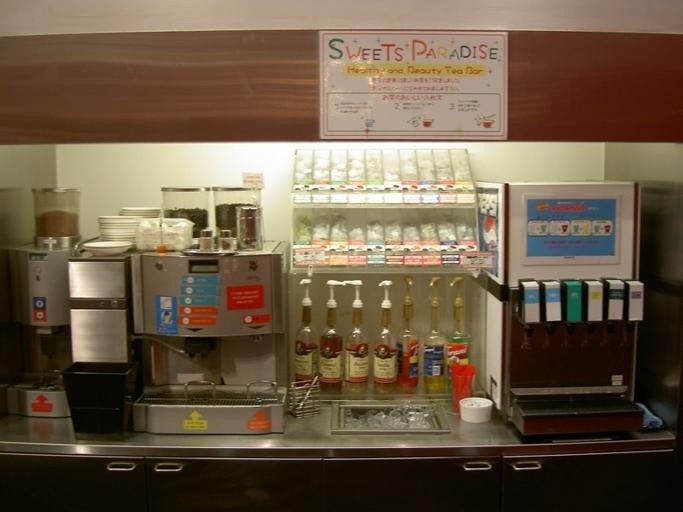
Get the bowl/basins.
[458,396,494,425]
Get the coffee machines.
[131,240,292,435]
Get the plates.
[79,207,162,255]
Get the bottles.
[198,229,214,248]
[219,230,235,253]
[237,206,268,254]
[159,186,262,244]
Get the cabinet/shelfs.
[324,449,683,512]
[0,452,324,512]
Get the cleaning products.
[294,276,472,400]
